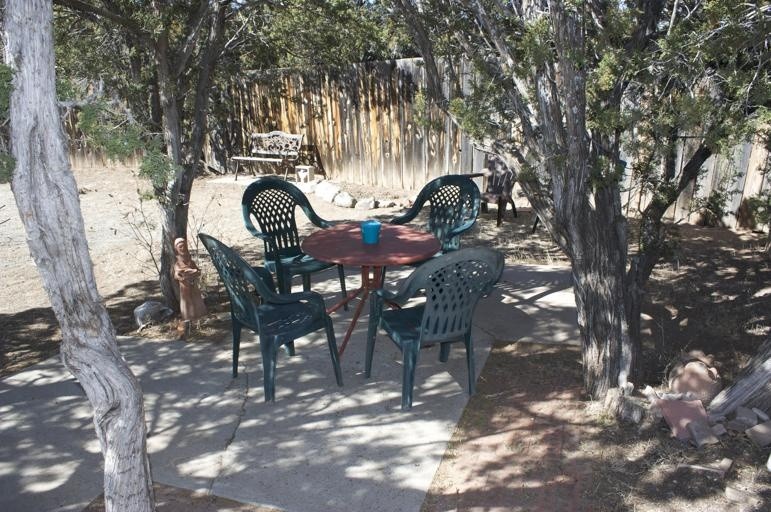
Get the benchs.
[231,131,304,181]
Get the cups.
[360,219,381,244]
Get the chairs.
[364,246,505,412]
[380,174,482,291]
[460,138,523,227]
[242,177,348,313]
[199,232,344,403]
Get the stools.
[294,165,316,183]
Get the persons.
[173,238,207,333]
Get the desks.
[301,221,441,356]
